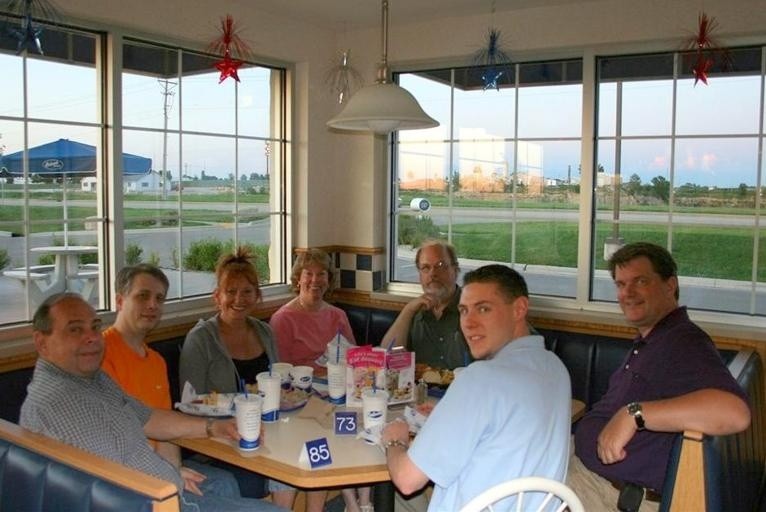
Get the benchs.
[560,350,754,507]
[337,303,734,431]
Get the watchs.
[383,439,408,458]
[205,417,214,437]
[624,401,646,433]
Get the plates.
[179,392,242,419]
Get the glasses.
[418,261,448,273]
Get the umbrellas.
[0,140,152,246]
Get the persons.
[270,249,356,376]
[99,265,207,495]
[380,265,572,512]
[562,242,751,512]
[16,293,292,511]
[381,242,539,371]
[178,249,275,397]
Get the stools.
[3,271,48,310]
[81,263,101,272]
[11,264,55,285]
[68,271,102,306]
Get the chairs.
[0,420,184,512]
[458,477,584,512]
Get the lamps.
[325,3,438,138]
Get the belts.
[611,479,661,502]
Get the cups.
[361,390,389,443]
[233,394,262,451]
[290,366,313,396]
[271,363,292,394]
[256,372,281,423]
[327,360,346,406]
